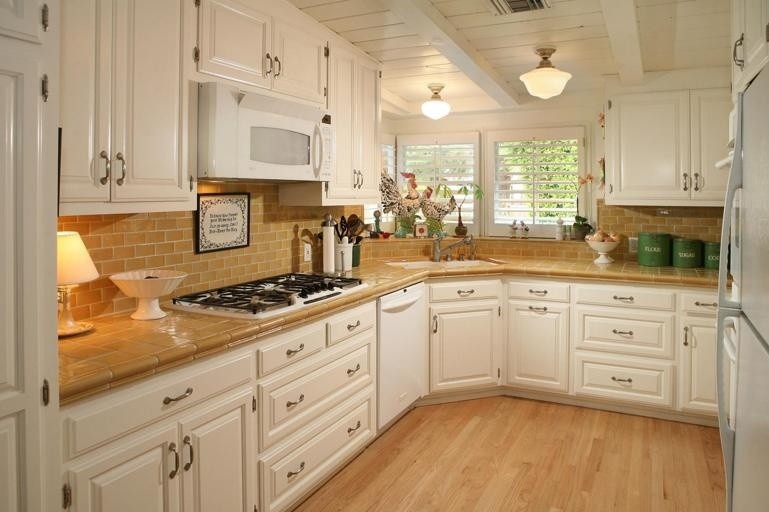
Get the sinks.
[384,257,510,270]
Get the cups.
[337,271,353,278]
[335,245,353,271]
[353,245,360,267]
[704,241,720,269]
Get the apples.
[585,230,620,242]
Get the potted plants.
[432,180,485,237]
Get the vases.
[573,224,591,240]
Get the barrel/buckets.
[352,243,362,267]
[703,241,730,269]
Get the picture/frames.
[194,192,252,253]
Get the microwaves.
[197,79,335,184]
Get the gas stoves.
[172,272,367,320]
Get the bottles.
[555,217,564,241]
[562,224,566,241]
[566,225,571,242]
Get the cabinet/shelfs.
[603,65,737,209]
[569,282,674,423]
[276,37,383,209]
[194,1,329,110]
[47,344,245,512]
[61,0,199,216]
[505,278,568,406]
[674,287,729,429]
[729,0,769,91]
[430,279,505,406]
[247,300,375,512]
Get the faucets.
[432,235,472,262]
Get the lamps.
[56,228,106,339]
[419,83,452,121]
[516,46,577,101]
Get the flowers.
[569,174,595,229]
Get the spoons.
[332,213,364,244]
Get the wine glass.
[108,269,190,320]
[586,240,621,265]
